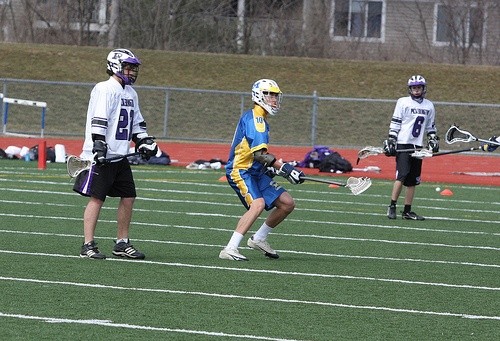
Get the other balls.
[436,187,440,192]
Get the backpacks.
[29,144,39,160]
[45,146,55,162]
[301,146,352,173]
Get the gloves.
[383,137,396,157]
[427,133,440,153]
[278,163,306,185]
[136,137,166,161]
[91,140,110,168]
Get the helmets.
[106,48,141,85]
[251,79,282,116]
[408,75,426,103]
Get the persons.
[483,136,500,151]
[211,79,306,261]
[72,47,159,259]
[383,74,441,221]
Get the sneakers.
[218,249,248,262]
[79,241,107,260]
[247,235,280,259]
[387,205,397,219]
[112,238,145,259]
[402,212,425,220]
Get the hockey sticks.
[445,125,500,146]
[300,176,372,196]
[358,144,427,159]
[67,151,144,178]
[411,145,481,158]
